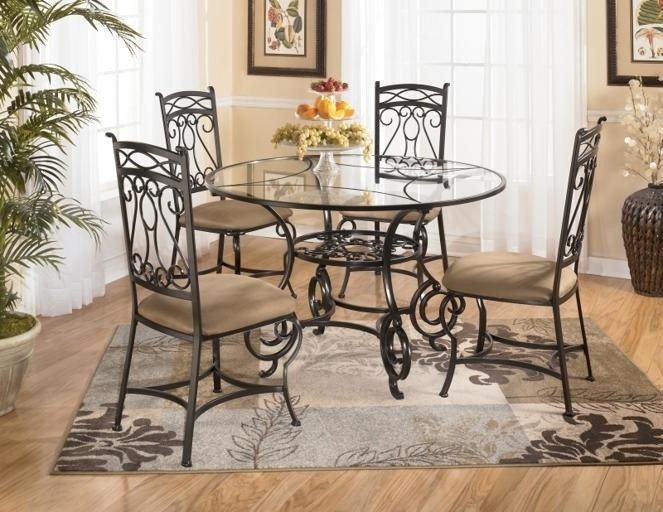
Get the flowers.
[624,73,663,188]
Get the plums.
[311,76,349,92]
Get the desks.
[204,154,507,400]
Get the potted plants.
[0,0,145,425]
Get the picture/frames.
[603,1,663,86]
[243,1,329,81]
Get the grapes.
[271,125,371,161]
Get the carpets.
[48,325,663,473]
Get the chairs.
[339,79,448,304]
[438,115,608,416]
[103,133,302,466]
[155,86,297,299]
